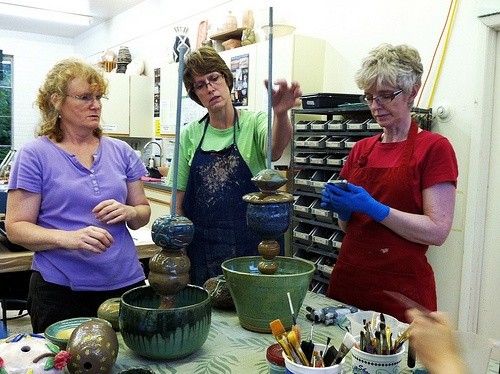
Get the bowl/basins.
[117,283,213,357]
[261,25,297,42]
[158,167,170,176]
[44,316,111,346]
[221,254,317,331]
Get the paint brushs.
[270,292,355,368]
[355,312,418,355]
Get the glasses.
[64,95,108,105]
[193,74,225,92]
[359,90,403,105]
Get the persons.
[3,59,151,335]
[164,45,302,288]
[405,308,471,374]
[319,43,459,325]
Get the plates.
[115,366,155,374]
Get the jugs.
[347,311,417,368]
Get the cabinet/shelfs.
[290,107,432,298]
[101,74,154,138]
[161,44,278,135]
[127,187,172,243]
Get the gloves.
[325,183,389,223]
[321,188,353,221]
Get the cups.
[266,344,286,374]
[351,334,405,374]
[283,343,346,374]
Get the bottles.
[243,9,255,28]
[223,10,237,29]
[149,158,154,167]
[173,26,191,62]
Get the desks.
[112,291,500,374]
